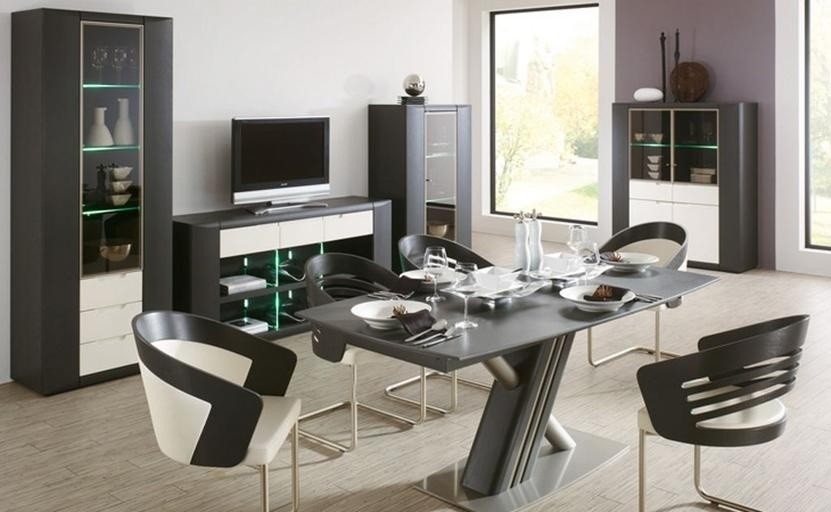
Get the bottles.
[88,97,135,147]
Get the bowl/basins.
[99,238,131,260]
[633,130,665,143]
[543,251,582,271]
[103,164,133,208]
[644,153,666,179]
[349,299,431,331]
[472,265,518,290]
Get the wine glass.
[577,240,600,284]
[568,224,588,256]
[90,46,130,87]
[450,261,481,330]
[421,241,447,304]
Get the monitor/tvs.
[230,117,330,214]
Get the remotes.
[301,203,328,208]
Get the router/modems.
[219,274,266,296]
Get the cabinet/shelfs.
[11,7,172,398]
[612,102,758,273]
[172,196,393,341]
[369,104,471,275]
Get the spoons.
[405,316,449,344]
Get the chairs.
[636,314,811,512]
[132,310,301,512]
[290,252,427,453]
[587,222,687,368]
[385,233,492,414]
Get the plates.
[559,284,635,313]
[600,251,659,274]
[401,268,466,284]
